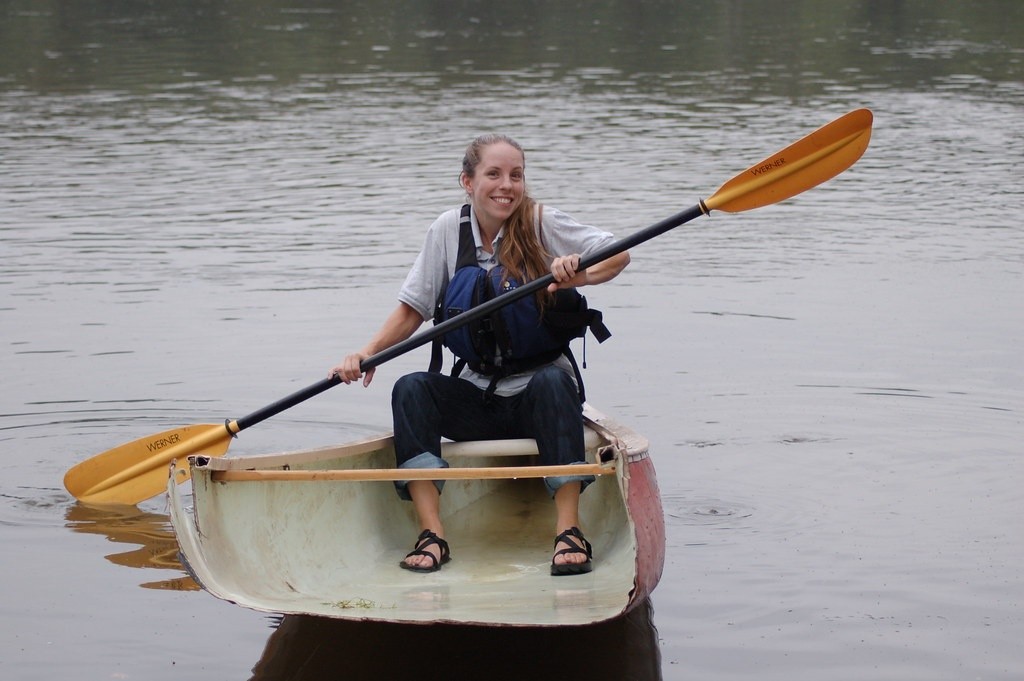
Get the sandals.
[551,526,593,575]
[399,529,451,573]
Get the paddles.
[64,107,874,505]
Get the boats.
[246,595,663,681]
[164,403,667,624]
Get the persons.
[327,132,632,576]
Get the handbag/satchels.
[440,266,578,376]
